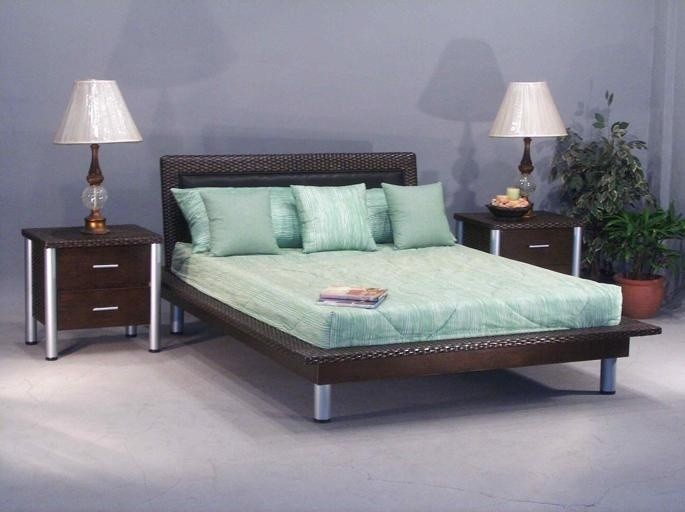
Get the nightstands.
[21,222,166,360]
[451,207,583,280]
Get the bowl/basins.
[484,204,533,222]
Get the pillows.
[380,175,459,250]
[168,180,397,254]
[198,185,284,259]
[289,180,379,256]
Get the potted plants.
[595,196,685,320]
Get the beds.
[155,144,666,429]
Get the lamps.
[486,78,570,218]
[50,76,147,235]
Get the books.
[316,284,389,309]
